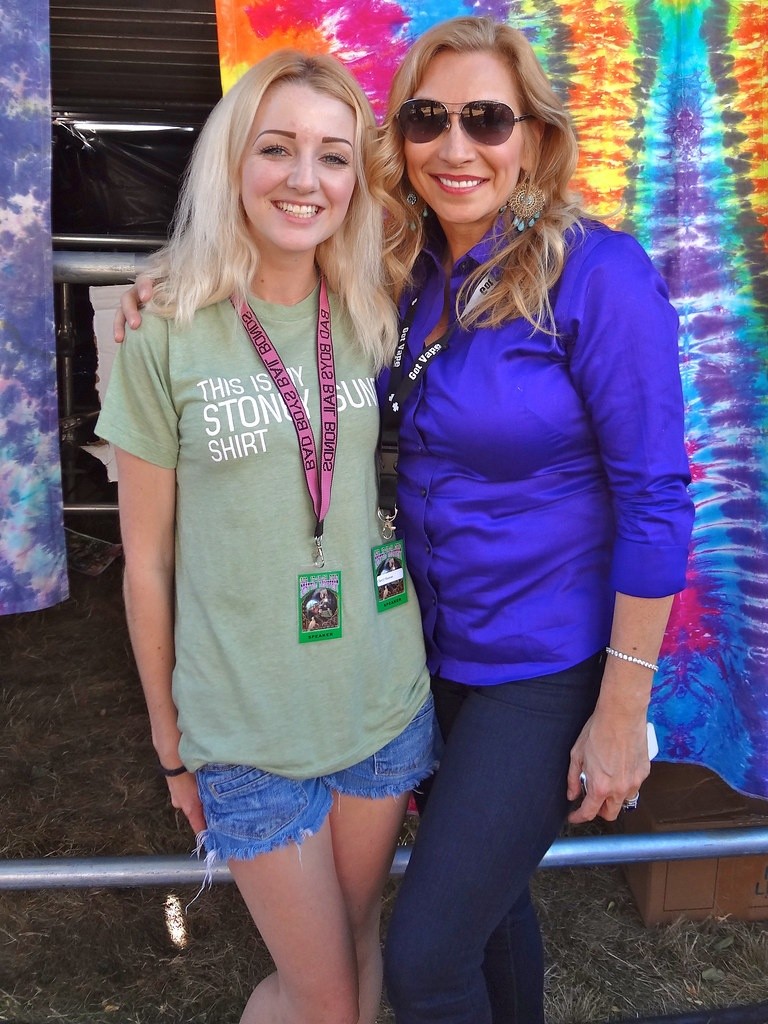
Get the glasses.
[394,99,535,146]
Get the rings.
[621,790,640,813]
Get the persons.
[95,50,438,1024]
[110,15,696,1024]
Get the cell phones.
[581,721,658,798]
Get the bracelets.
[606,647,660,673]
[161,765,188,778]
